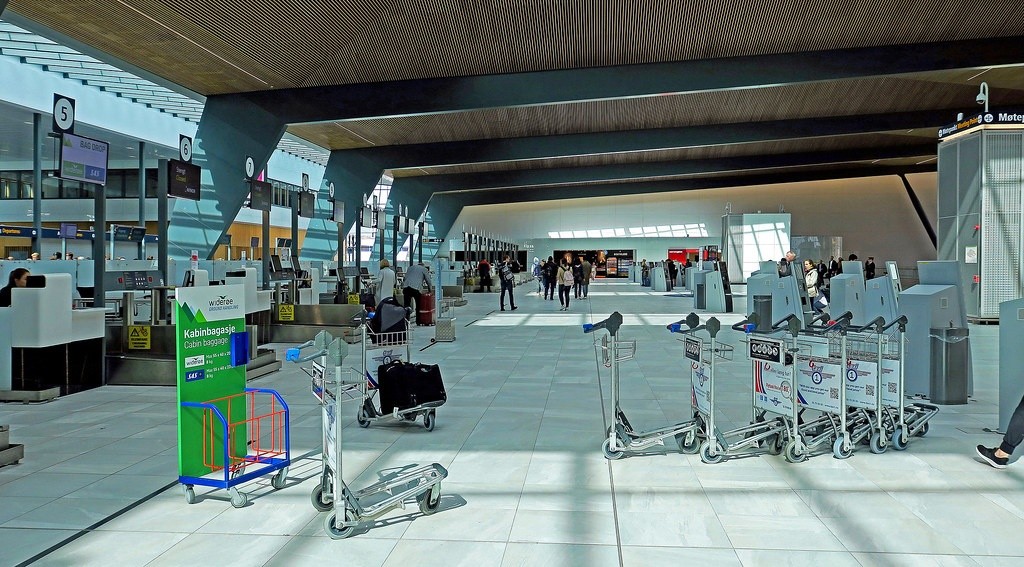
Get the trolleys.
[666,312,787,465]
[732,312,940,464]
[286,329,447,539]
[178,386,290,509]
[582,311,702,460]
[348,308,444,430]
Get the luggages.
[420,292,435,326]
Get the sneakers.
[976,444,1009,468]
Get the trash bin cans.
[696,283,704,309]
[931,327,970,404]
[753,295,772,332]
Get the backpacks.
[368,297,407,346]
[559,266,574,287]
[501,262,515,288]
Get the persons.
[499,255,517,311]
[778,251,876,314]
[976,397,1024,469]
[0,268,31,307]
[367,259,395,309]
[531,256,597,311]
[478,258,492,293]
[0,251,154,260]
[661,259,692,286]
[401,263,432,326]
[640,259,649,286]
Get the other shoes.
[559,305,565,310]
[511,306,519,311]
[501,308,505,311]
[814,311,824,317]
[565,307,569,310]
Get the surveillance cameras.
[976,93,986,104]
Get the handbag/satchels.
[377,359,447,414]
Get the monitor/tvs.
[397,267,403,277]
[336,268,345,281]
[271,255,282,273]
[361,268,369,279]
[290,255,301,271]
[182,270,194,287]
[890,264,897,279]
